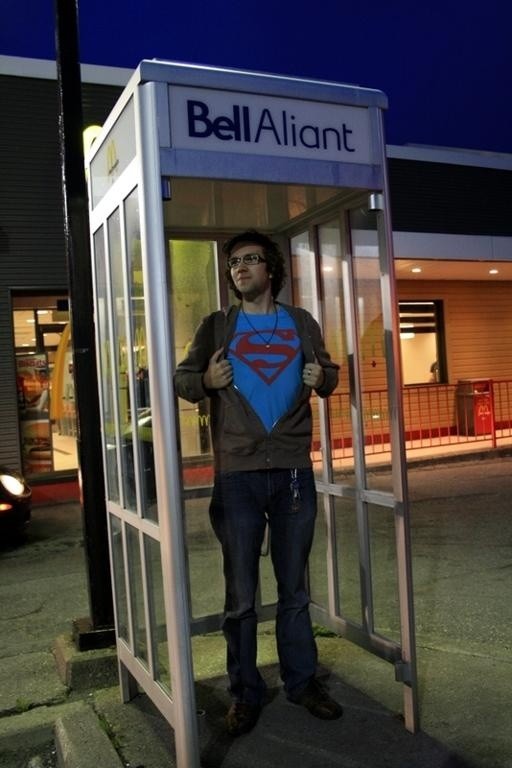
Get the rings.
[308,369,312,376]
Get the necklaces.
[241,304,278,348]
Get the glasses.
[227,254,265,268]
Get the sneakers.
[224,703,255,734]
[297,681,343,720]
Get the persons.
[172,228,344,736]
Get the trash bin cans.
[124,407,155,506]
[455,378,492,435]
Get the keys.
[290,478,301,511]
